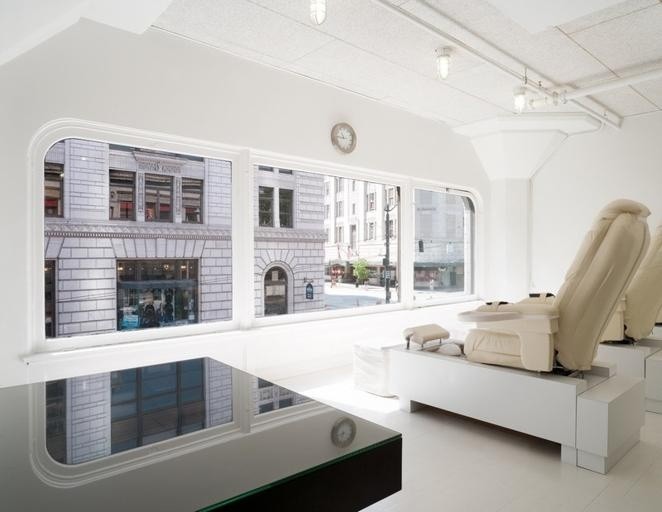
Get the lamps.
[511,86,526,117]
[307,0,327,27]
[433,43,454,82]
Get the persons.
[131,288,196,330]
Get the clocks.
[331,417,357,448]
[330,122,357,155]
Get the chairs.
[459,197,662,374]
